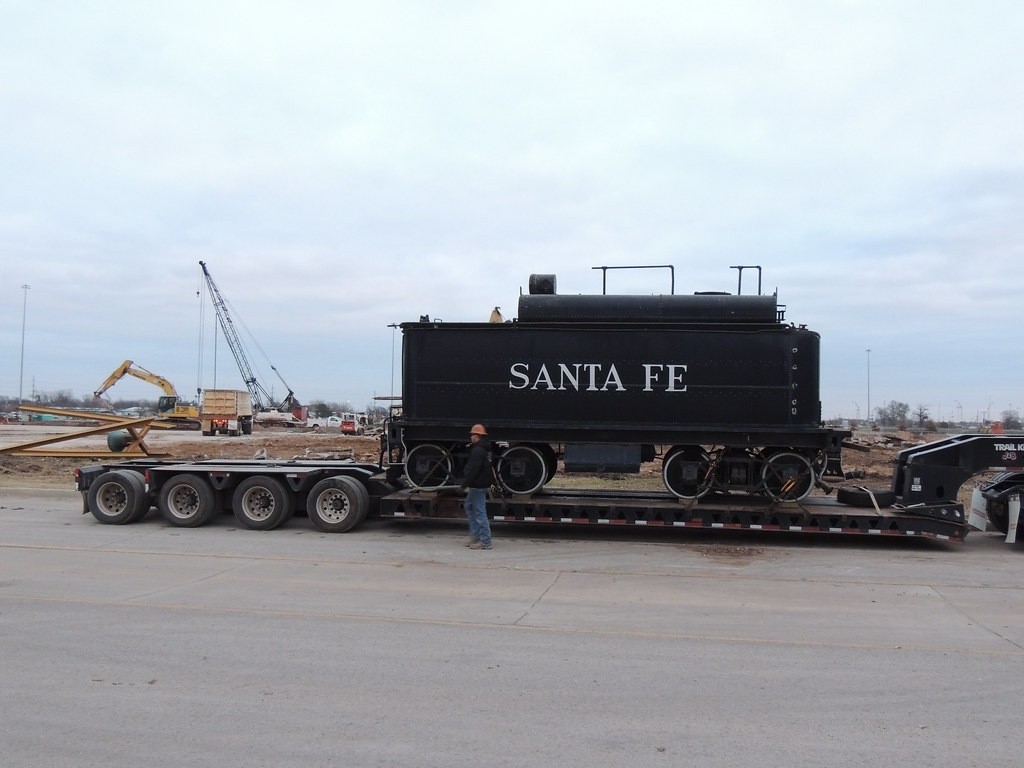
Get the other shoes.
[470,541,492,549]
[466,539,481,546]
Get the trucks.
[201,390,254,437]
[341,420,364,436]
[307,416,342,431]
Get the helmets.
[469,424,487,435]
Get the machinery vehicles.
[91,359,202,430]
[196,260,308,426]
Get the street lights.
[865,348,871,419]
[19,284,30,405]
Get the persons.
[461,424,493,550]
